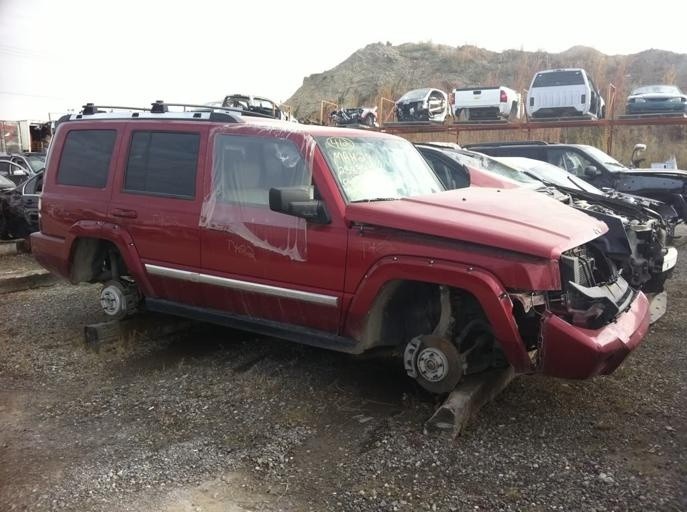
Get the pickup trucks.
[453,87,521,122]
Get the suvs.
[525,68,606,119]
[27,94,651,394]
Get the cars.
[0,117,55,240]
[626,86,687,114]
[329,104,378,126]
[396,89,449,122]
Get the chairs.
[224,162,269,205]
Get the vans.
[222,95,297,121]
